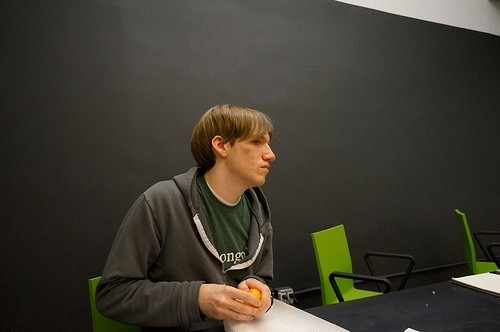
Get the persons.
[94,103,276,331]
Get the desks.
[195,271,500,332]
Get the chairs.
[311,223,416,306]
[456,210,500,274]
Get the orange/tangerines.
[235,288,262,308]
